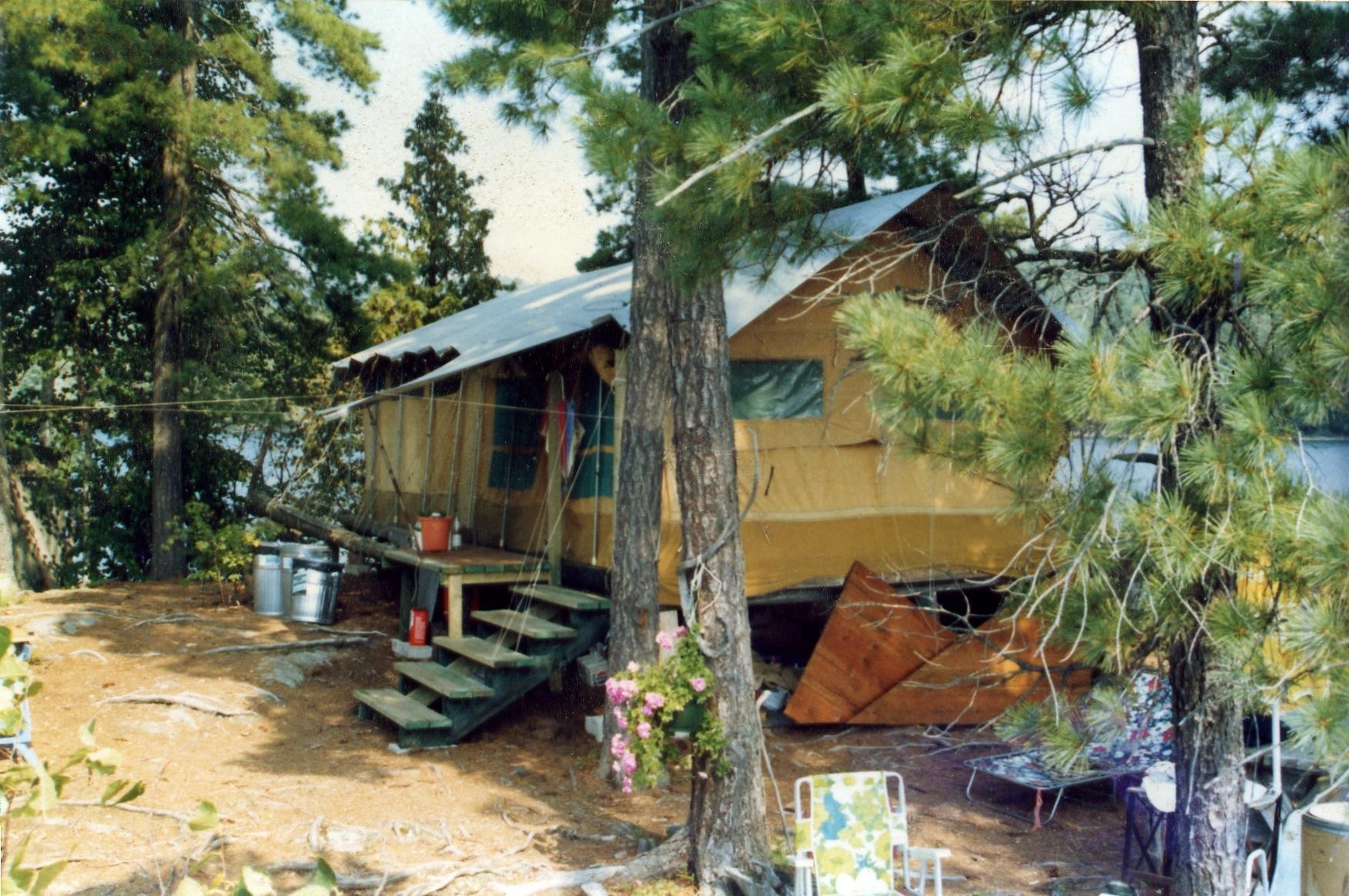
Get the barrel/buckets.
[1300,801,1348,896]
[251,541,350,624]
[419,516,453,552]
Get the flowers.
[604,627,733,795]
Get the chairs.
[1121,779,1284,892]
[787,769,951,896]
[964,666,1174,827]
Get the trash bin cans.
[281,545,333,618]
[253,542,300,617]
[288,558,344,626]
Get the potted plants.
[665,697,704,730]
